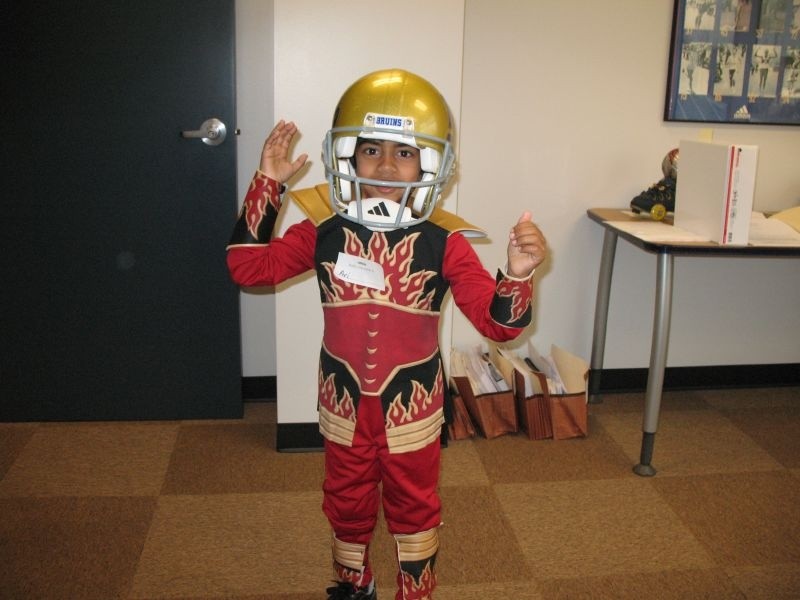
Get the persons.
[680,0,800,98]
[226,68,546,600]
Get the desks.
[586,207,800,476]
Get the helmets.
[321,68,453,233]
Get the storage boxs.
[673,140,758,248]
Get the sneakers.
[326,581,376,600]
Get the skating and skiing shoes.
[630,174,678,220]
